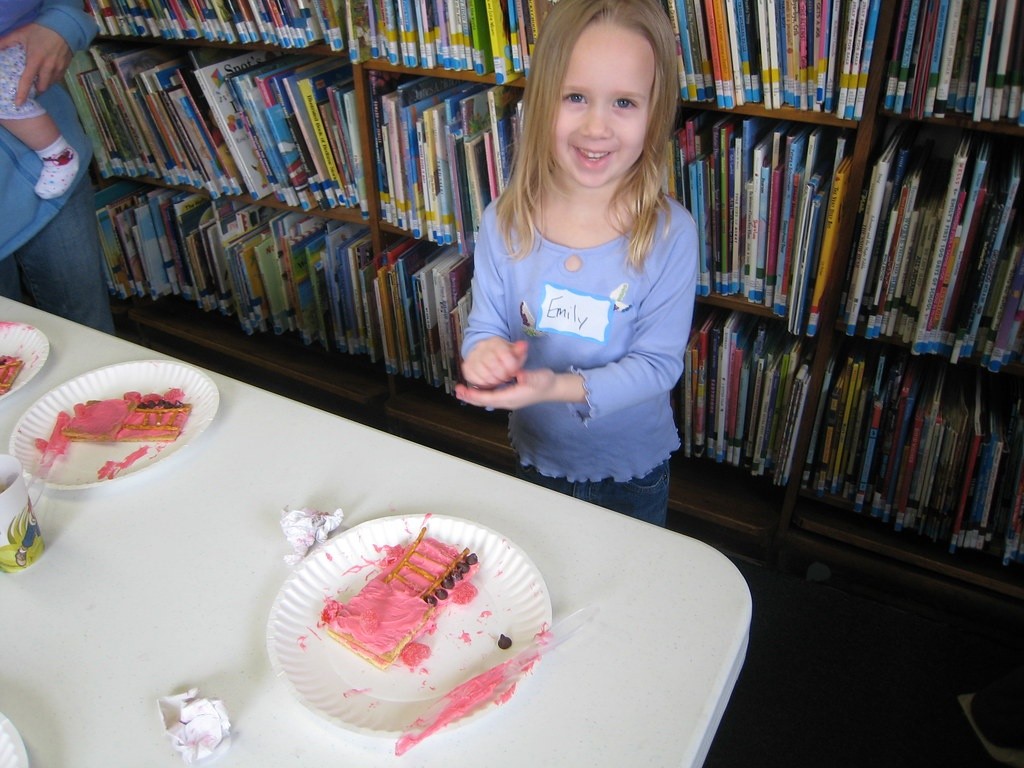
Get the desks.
[0,295,753,768]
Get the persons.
[0,0,115,337]
[455,1,700,526]
[0,0,80,199]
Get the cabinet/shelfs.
[57,0,1024,647]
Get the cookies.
[59,399,192,442]
[326,527,470,671]
[0,356,23,394]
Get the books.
[79,0,1023,564]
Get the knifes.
[26,410,71,508]
[395,606,597,755]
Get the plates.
[0,320,50,402]
[8,359,220,491]
[265,513,553,739]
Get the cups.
[0,454,46,574]
